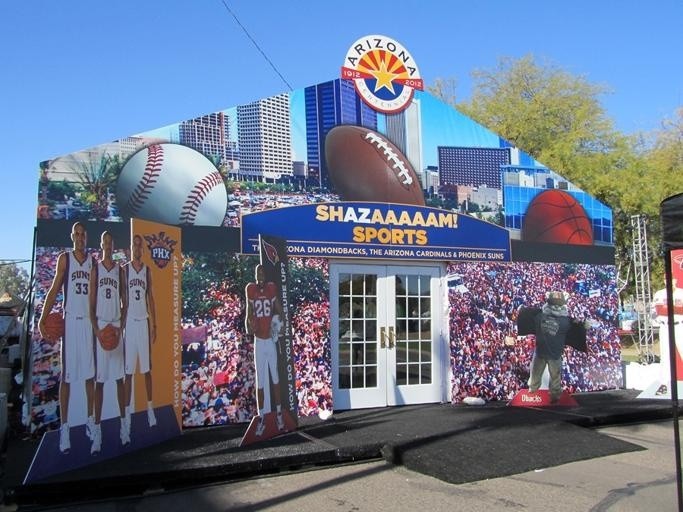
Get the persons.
[224,190,341,226]
[445,262,622,404]
[180,256,334,424]
[405,308,430,330]
[458,199,505,228]
[32,192,157,456]
[654,272,683,395]
[351,310,364,371]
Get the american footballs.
[325,125,424,206]
[248,316,260,332]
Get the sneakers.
[276,413,286,432]
[148,401,158,427]
[85,416,103,454]
[59,422,71,456]
[119,413,131,446]
[255,417,267,436]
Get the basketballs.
[45,313,65,343]
[521,190,593,245]
[101,322,118,350]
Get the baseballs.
[116,143,227,226]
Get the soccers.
[271,315,285,332]
